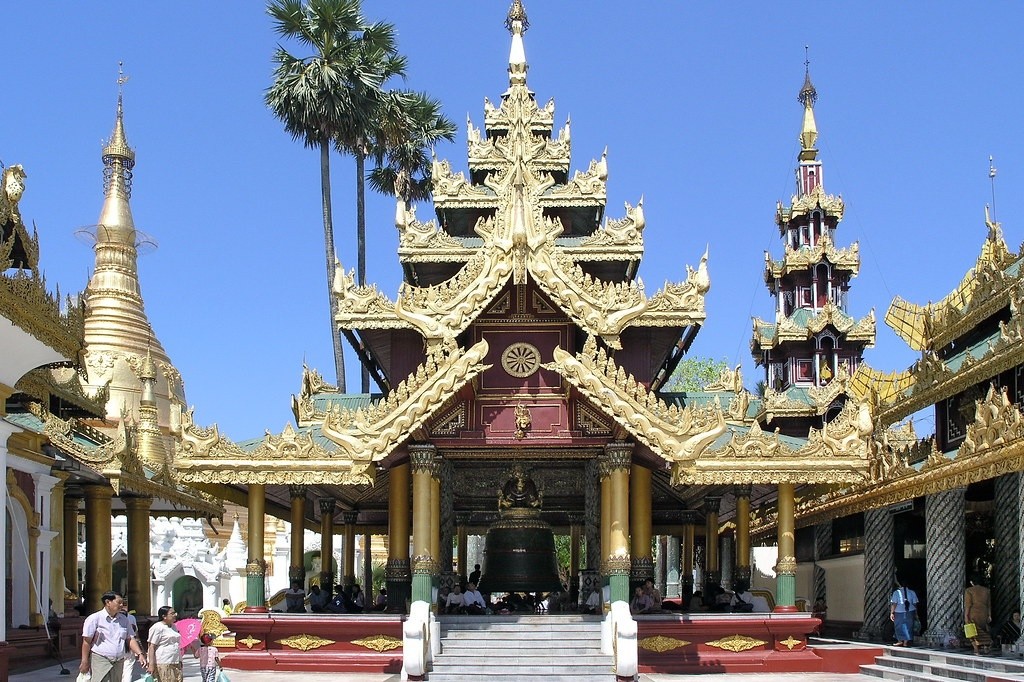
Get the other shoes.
[893,642,907,647]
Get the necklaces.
[964,572,992,654]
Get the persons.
[547,591,559,611]
[223,599,232,617]
[376,589,387,609]
[191,634,223,682]
[689,591,709,610]
[309,585,351,613]
[630,577,662,614]
[285,581,305,612]
[813,598,828,637]
[146,606,184,682]
[440,564,534,615]
[890,579,919,647]
[48,598,61,631]
[79,591,146,682]
[350,584,364,608]
[996,611,1021,645]
[586,582,600,614]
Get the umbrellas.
[173,618,202,648]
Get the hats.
[200,634,214,646]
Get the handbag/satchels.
[913,620,921,632]
[964,623,978,638]
[216,671,230,682]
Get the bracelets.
[138,653,144,658]
